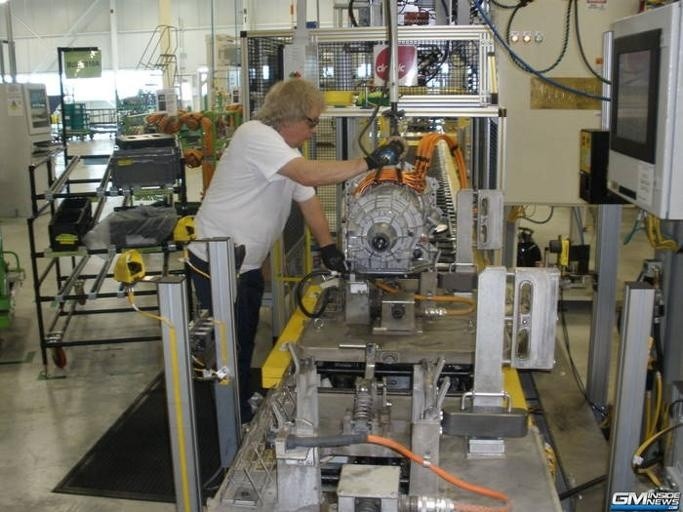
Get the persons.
[185,79,390,422]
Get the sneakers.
[241,391,264,440]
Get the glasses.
[301,114,320,129]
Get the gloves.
[363,143,400,171]
[320,242,352,274]
